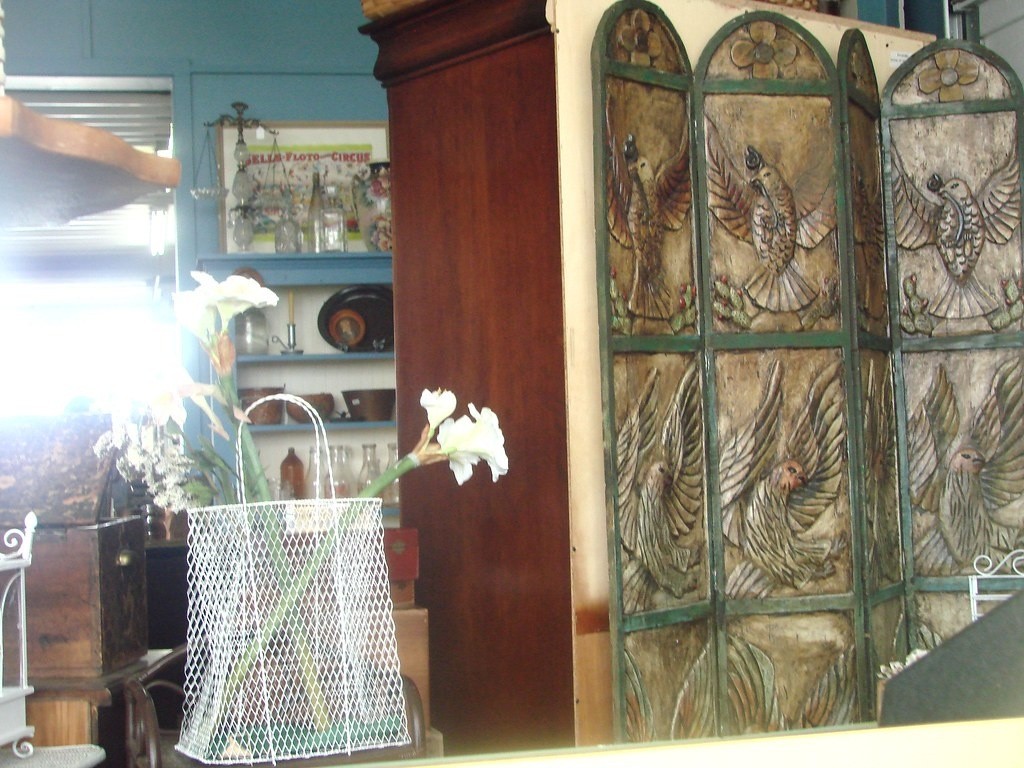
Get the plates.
[317,284,394,353]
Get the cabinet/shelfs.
[356,1,938,756]
[1,514,151,692]
[195,252,396,433]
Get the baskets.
[175,392,414,766]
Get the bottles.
[304,446,326,500]
[234,307,271,356]
[322,445,354,499]
[357,442,383,501]
[279,447,305,502]
[273,204,301,256]
[383,442,399,508]
[307,171,325,253]
[357,161,394,253]
[318,186,346,254]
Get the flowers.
[96,269,508,516]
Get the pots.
[286,392,333,424]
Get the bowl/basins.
[236,386,284,426]
[342,389,397,422]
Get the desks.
[24,649,174,768]
[0,745,107,767]
[392,607,430,757]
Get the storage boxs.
[170,394,413,765]
[237,385,285,426]
[341,389,396,421]
[285,393,334,424]
[390,581,416,611]
[382,528,421,582]
